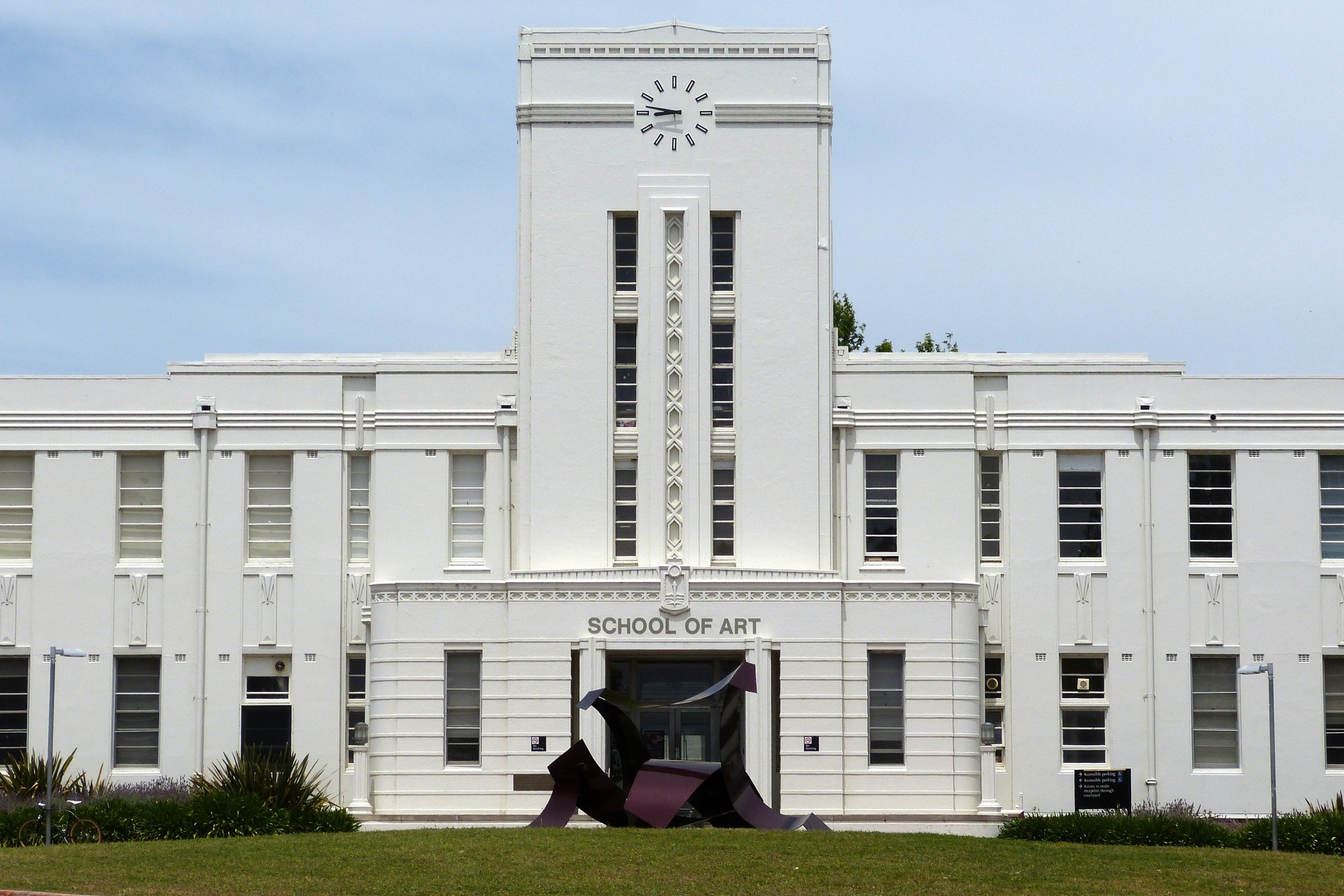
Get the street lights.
[45,645,87,847]
[1236,662,1278,852]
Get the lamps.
[354,722,368,746]
[980,723,995,746]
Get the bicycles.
[18,800,100,848]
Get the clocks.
[636,76,713,151]
